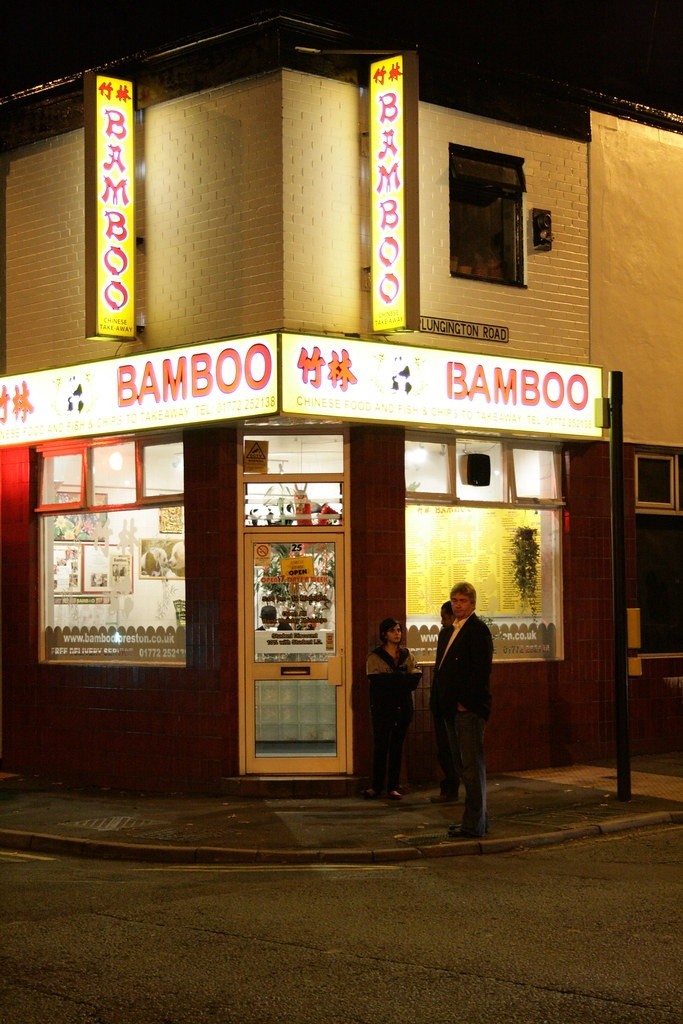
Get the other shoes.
[447,822,488,838]
[431,793,458,804]
[387,789,401,800]
[362,789,384,799]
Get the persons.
[364,619,422,800]
[428,581,493,839]
[255,607,296,662]
